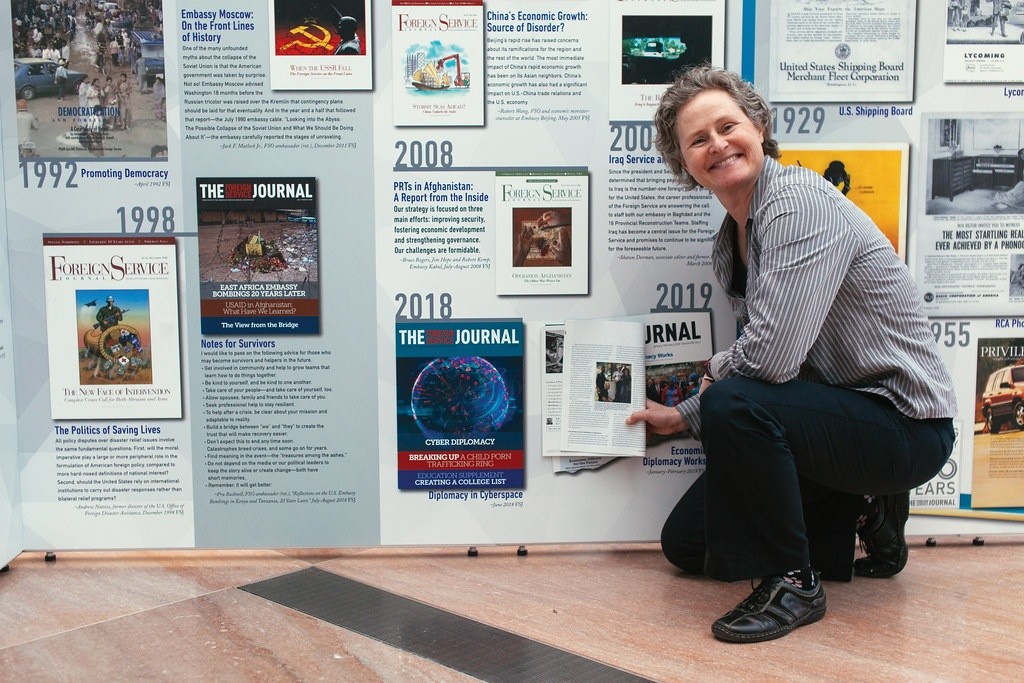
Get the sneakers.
[851,492,908,576]
[714,574,827,642]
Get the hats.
[151,146,162,157]
[58,60,65,66]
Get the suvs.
[980,364,1024,434]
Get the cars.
[13,57,88,102]
[103,18,132,43]
[95,0,125,22]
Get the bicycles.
[97,45,112,78]
[153,96,166,121]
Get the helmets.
[17,100,27,110]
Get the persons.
[626,67,958,643]
[647,372,699,407]
[1012,148,1024,186]
[596,365,631,402]
[986,0,1011,37]
[13,0,167,157]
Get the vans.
[127,28,164,80]
